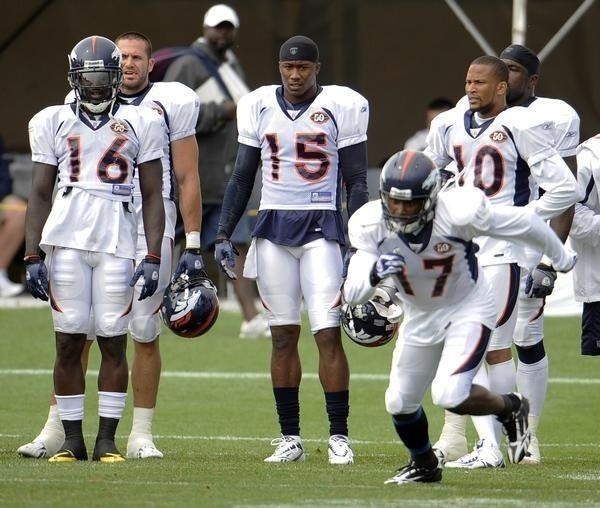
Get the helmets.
[378,150,438,233]
[68,35,123,113]
[339,288,402,347]
[159,276,219,339]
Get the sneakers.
[239,313,275,340]
[92,445,127,464]
[126,438,162,458]
[521,435,541,465]
[425,435,468,470]
[264,436,307,464]
[0,268,23,297]
[15,434,65,457]
[497,391,530,467]
[326,434,356,464]
[383,458,443,485]
[444,439,506,470]
[46,443,89,462]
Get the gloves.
[369,252,406,286]
[171,247,207,280]
[23,254,50,303]
[128,254,160,302]
[524,263,557,299]
[214,239,241,281]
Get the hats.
[203,3,240,30]
[279,35,319,64]
[498,45,541,75]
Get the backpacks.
[150,44,238,104]
